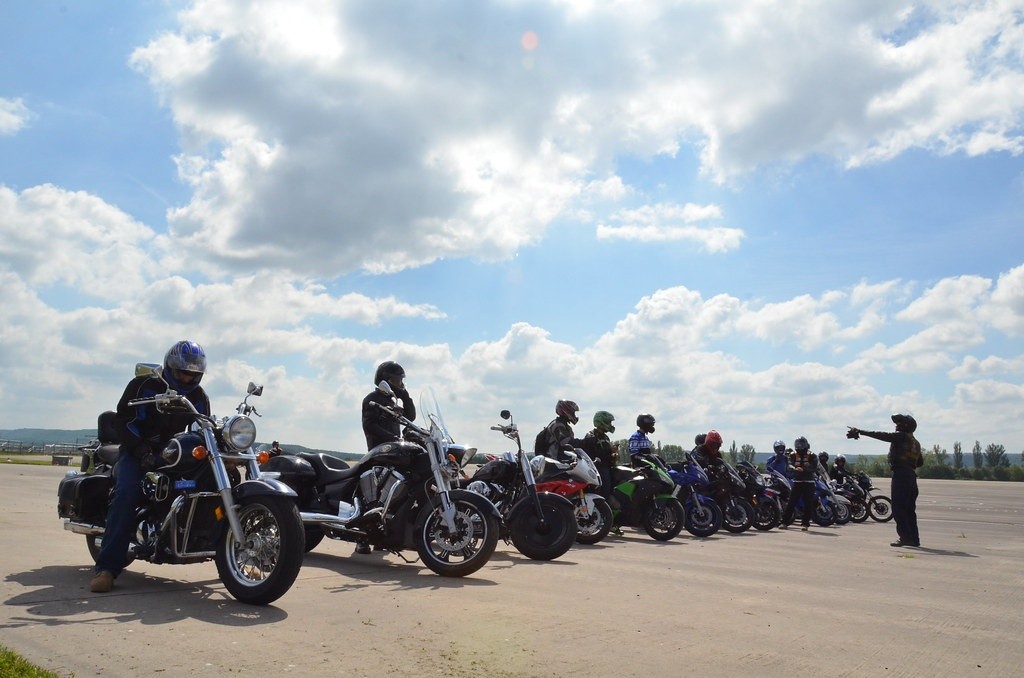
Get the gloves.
[846,429,859,440]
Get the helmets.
[637,413,656,433]
[818,450,830,463]
[695,433,708,446]
[556,399,579,425]
[794,436,810,454]
[705,430,724,455]
[593,410,616,433]
[164,340,206,395]
[773,440,786,455]
[835,454,846,468]
[891,414,917,433]
[374,360,406,399]
[785,448,793,454]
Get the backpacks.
[535,419,558,458]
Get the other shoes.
[801,525,808,531]
[612,526,624,536]
[90,570,114,592]
[778,524,788,529]
[354,542,372,554]
[890,536,920,547]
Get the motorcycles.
[810,475,836,526]
[852,470,893,522]
[610,448,684,542]
[834,474,871,523]
[259,380,505,577]
[57,363,307,605]
[763,465,797,528]
[452,410,579,562]
[824,477,852,525]
[656,450,723,538]
[531,443,613,545]
[734,460,780,530]
[703,457,759,533]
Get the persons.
[582,411,625,535]
[91,341,211,593]
[546,400,578,463]
[355,362,416,553]
[847,414,924,546]
[690,430,728,479]
[778,437,818,530]
[818,451,828,476]
[766,440,788,478]
[830,454,857,484]
[268,440,282,457]
[628,412,672,472]
